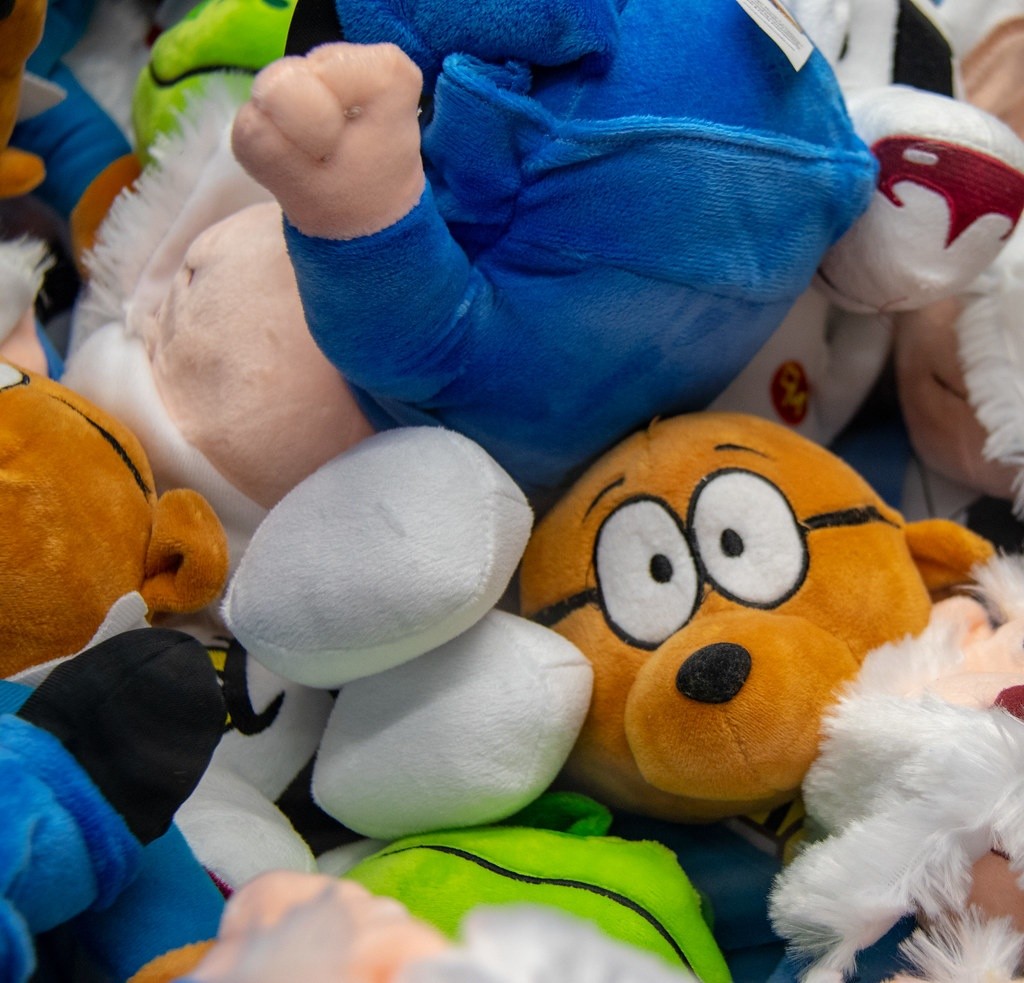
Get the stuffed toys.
[0,0,1024,983]
